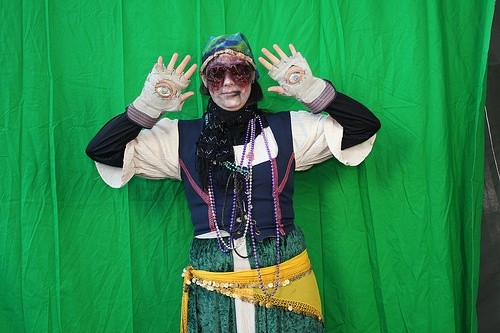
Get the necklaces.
[202,111,281,298]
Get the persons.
[85,33,381,332]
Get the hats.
[200,33,261,80]
